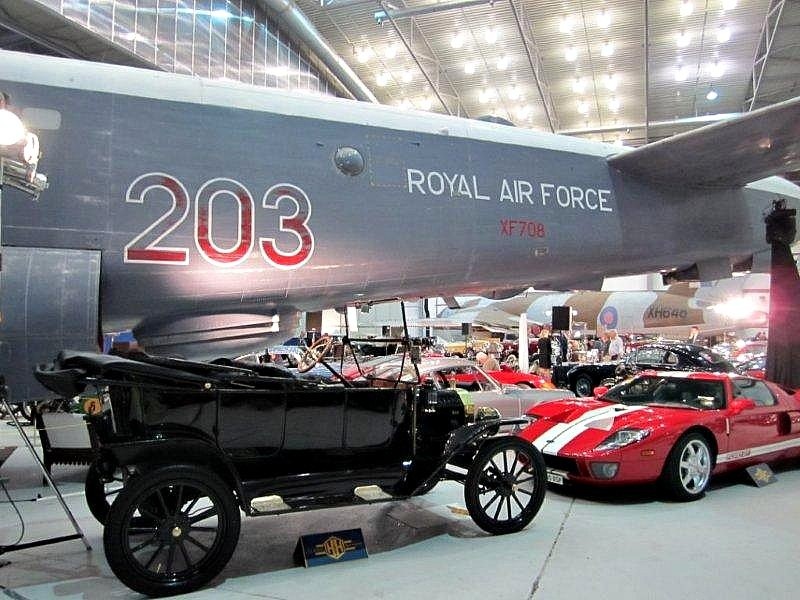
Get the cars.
[226,319,800,502]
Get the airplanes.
[358,271,769,350]
[0,45,800,421]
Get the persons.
[536,330,552,377]
[601,330,623,356]
[687,326,704,346]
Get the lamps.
[707,85,718,100]
